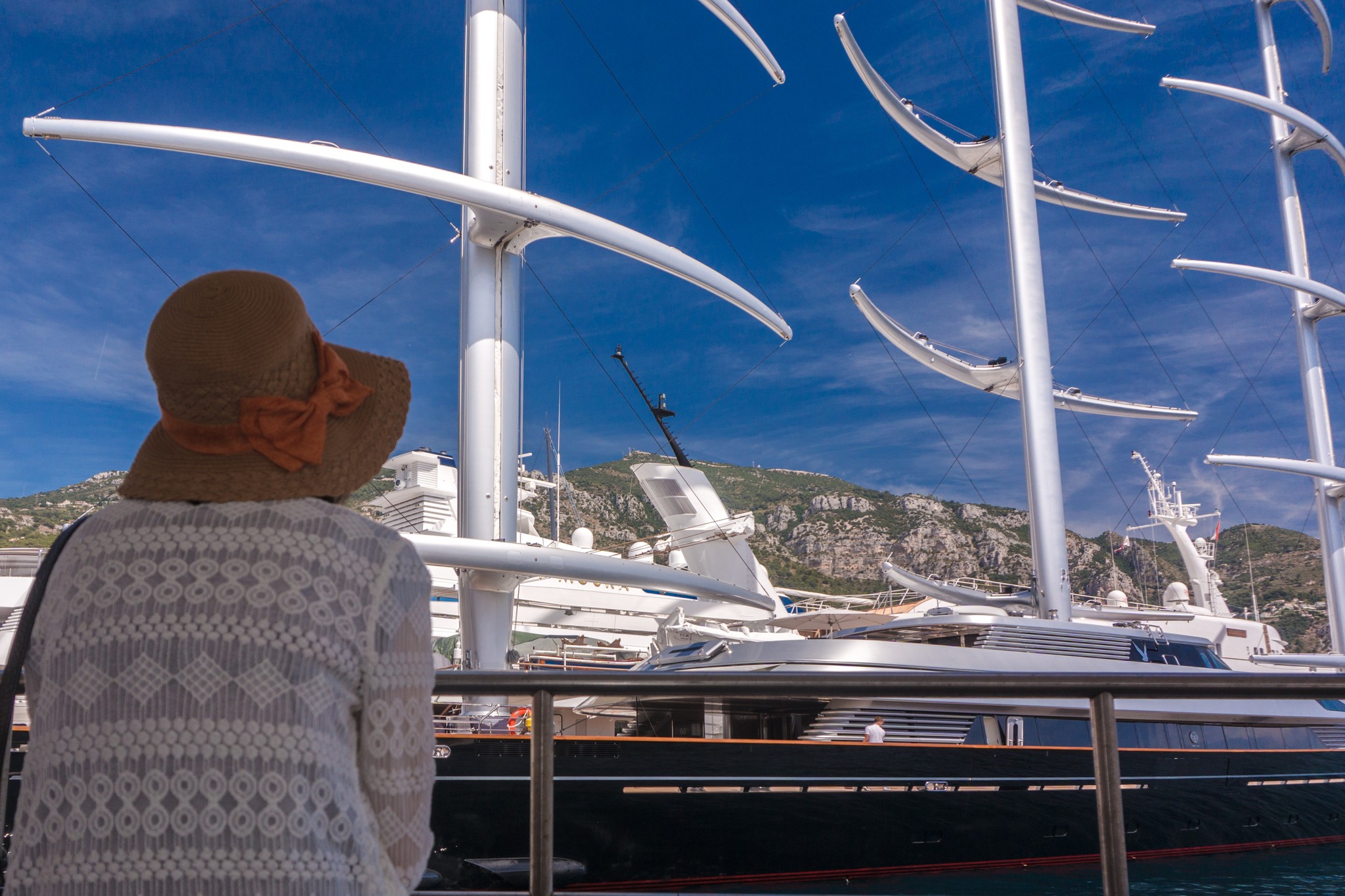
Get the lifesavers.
[507,708,533,737]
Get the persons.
[1,270,435,896]
[863,715,891,791]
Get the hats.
[117,269,412,502]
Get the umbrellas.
[765,607,900,639]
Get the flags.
[1210,520,1220,541]
[1113,535,1130,552]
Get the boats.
[1,0,1343,896]
